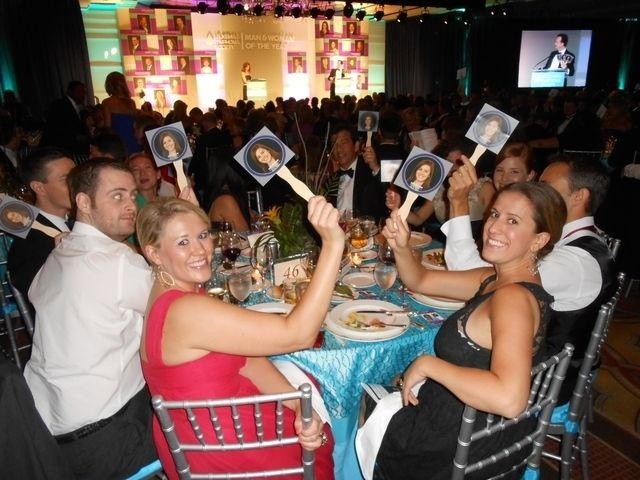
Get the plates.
[409,232,432,247]
[360,250,377,261]
[422,248,448,270]
[247,304,295,316]
[342,273,376,288]
[331,292,354,305]
[409,293,465,310]
[324,300,410,342]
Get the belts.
[55,401,130,446]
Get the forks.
[365,324,407,328]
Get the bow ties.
[556,52,562,55]
[565,116,574,119]
[339,168,354,178]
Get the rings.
[319,432,327,444]
[397,379,403,389]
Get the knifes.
[357,310,411,314]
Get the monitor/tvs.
[516,28,593,90]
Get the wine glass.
[379,242,396,265]
[374,263,397,301]
[250,245,274,291]
[345,209,375,249]
[248,191,263,222]
[229,273,252,309]
[211,219,234,238]
[223,234,242,274]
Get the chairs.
[605,233,621,260]
[0,232,34,368]
[152,384,316,480]
[451,343,575,480]
[541,273,626,479]
[0,358,162,480]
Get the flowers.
[249,201,309,270]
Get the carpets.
[501,290,640,480]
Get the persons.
[160,98,183,131]
[355,75,366,89]
[6,151,77,324]
[155,91,165,109]
[330,41,337,51]
[177,17,185,33]
[362,114,374,130]
[357,42,364,54]
[241,62,251,100]
[134,102,160,152]
[347,60,356,70]
[320,21,330,34]
[201,146,259,232]
[183,97,325,157]
[325,125,390,226]
[504,88,640,154]
[171,80,180,93]
[294,59,302,73]
[325,96,362,125]
[386,147,497,250]
[166,38,178,55]
[478,114,503,145]
[477,78,503,114]
[159,131,182,159]
[322,58,329,70]
[348,23,356,35]
[362,92,374,114]
[23,157,200,480]
[100,71,144,158]
[354,180,568,480]
[135,79,145,99]
[3,207,32,229]
[146,57,154,75]
[0,83,103,167]
[542,33,575,88]
[140,16,151,34]
[328,60,345,98]
[122,153,179,254]
[619,154,640,281]
[136,195,346,480]
[440,154,620,408]
[131,37,140,54]
[493,143,535,191]
[407,159,435,191]
[250,144,281,172]
[374,85,477,147]
[202,58,211,73]
[181,58,190,74]
